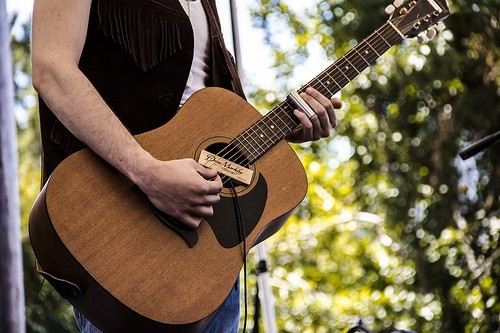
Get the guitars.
[27,0,450,332]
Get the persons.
[28,1,344,333]
